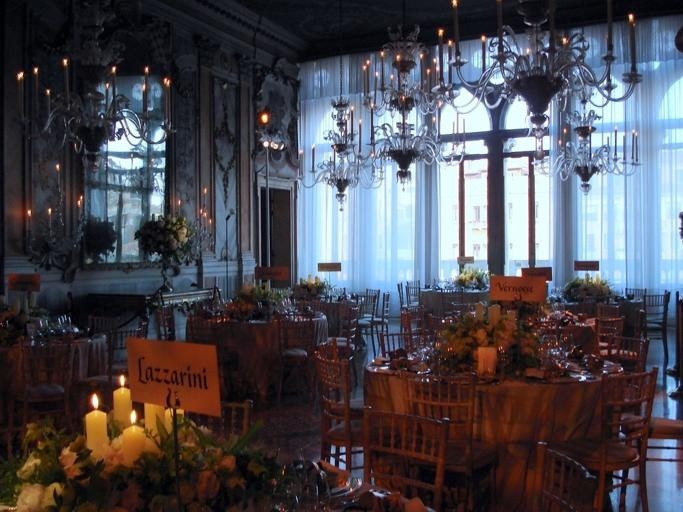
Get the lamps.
[17,0,176,174]
[294,1,638,214]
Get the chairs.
[0,280,683,512]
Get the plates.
[332,477,362,499]
